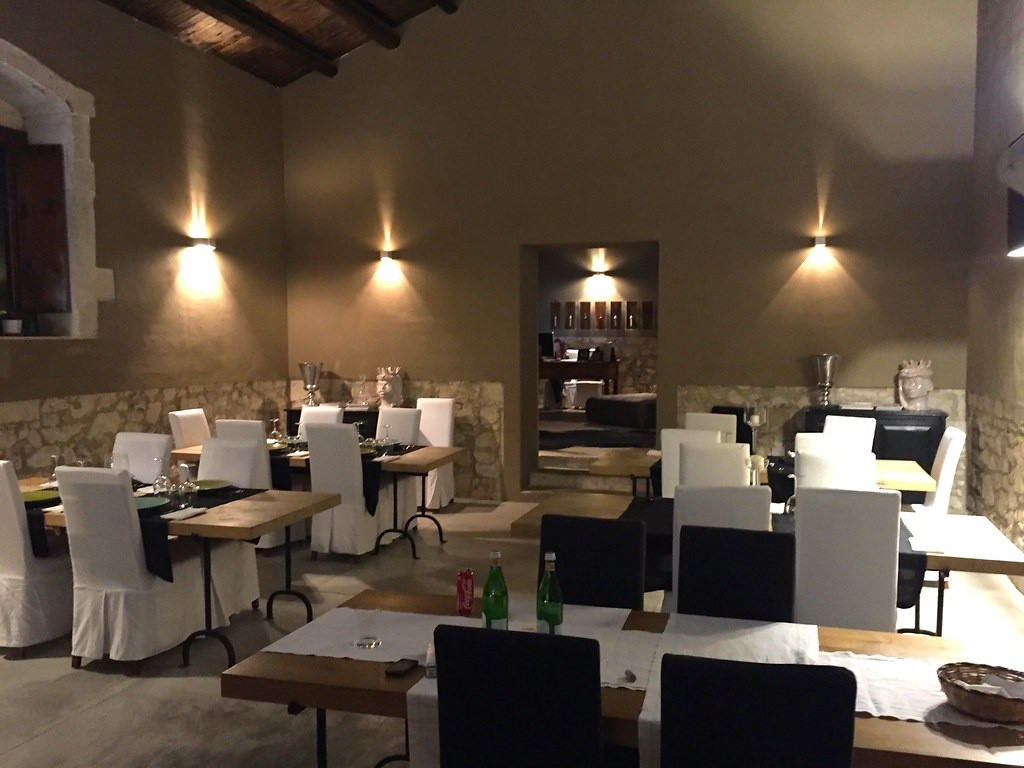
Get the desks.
[628,448,938,510]
[17,477,341,672]
[617,496,1023,636]
[221,589,1024,768]
[169,439,465,559]
[283,406,378,438]
[541,359,620,395]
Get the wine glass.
[152,456,172,498]
[296,423,304,443]
[48,454,63,484]
[383,425,393,446]
[785,473,807,517]
[298,362,323,406]
[268,418,283,440]
[353,422,365,446]
[815,354,837,407]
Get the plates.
[192,479,232,489]
[20,491,61,506]
[361,448,375,454]
[377,442,401,446]
[134,497,170,509]
[267,442,287,449]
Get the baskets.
[937,662,1024,725]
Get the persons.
[549,339,569,410]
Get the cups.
[751,467,768,483]
[104,452,129,474]
[354,603,384,648]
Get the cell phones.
[385,658,418,677]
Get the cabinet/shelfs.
[805,405,949,505]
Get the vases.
[298,361,324,406]
[814,352,842,408]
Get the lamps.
[381,250,394,262]
[814,236,826,247]
[592,271,605,277]
[1006,185,1024,259]
[191,237,216,250]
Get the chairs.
[0,398,457,670]
[538,513,647,610]
[660,416,966,768]
[432,624,606,768]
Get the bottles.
[536,552,563,636]
[553,338,562,360]
[568,314,573,328]
[583,313,588,328]
[613,314,618,327]
[0,310,22,334]
[480,551,509,631]
[553,315,557,327]
[629,314,633,327]
[598,313,603,327]
[426,643,436,677]
[168,464,196,510]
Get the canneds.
[455,568,474,615]
